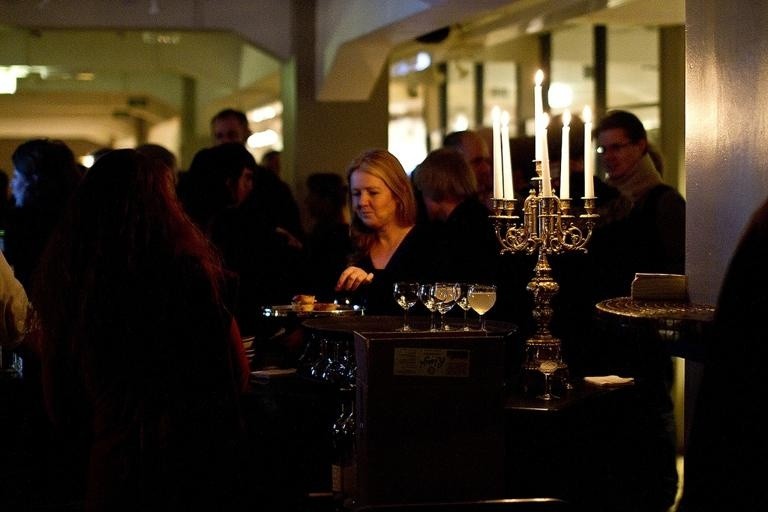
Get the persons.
[508,133,560,246]
[1,106,504,511]
[585,108,684,512]
[542,109,632,223]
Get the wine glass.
[536,359,559,402]
[392,281,498,331]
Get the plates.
[581,374,635,386]
[260,305,367,319]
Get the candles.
[559,111,572,199]
[501,111,515,199]
[490,105,504,199]
[542,112,552,195]
[582,106,595,198]
[533,68,545,160]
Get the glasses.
[595,140,634,152]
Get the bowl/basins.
[240,335,255,364]
